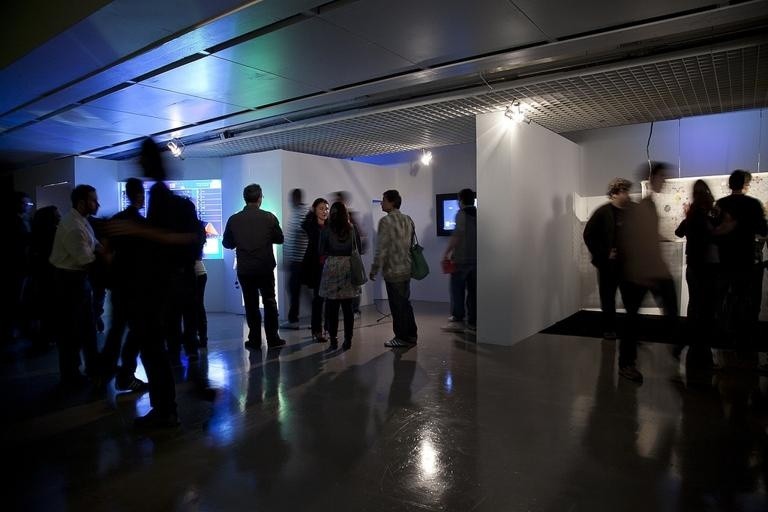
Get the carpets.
[540,309,768,350]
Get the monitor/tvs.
[436,193,477,236]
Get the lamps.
[166,137,184,158]
[418,148,433,166]
[504,97,524,124]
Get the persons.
[618,160,683,383]
[582,175,639,340]
[716,168,767,363]
[672,179,735,360]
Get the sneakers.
[244,339,262,348]
[618,362,643,385]
[384,337,411,347]
[439,315,477,337]
[114,308,210,396]
[133,408,181,431]
[267,339,286,348]
[353,312,361,320]
[326,339,353,353]
[279,320,300,330]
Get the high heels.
[311,325,331,342]
